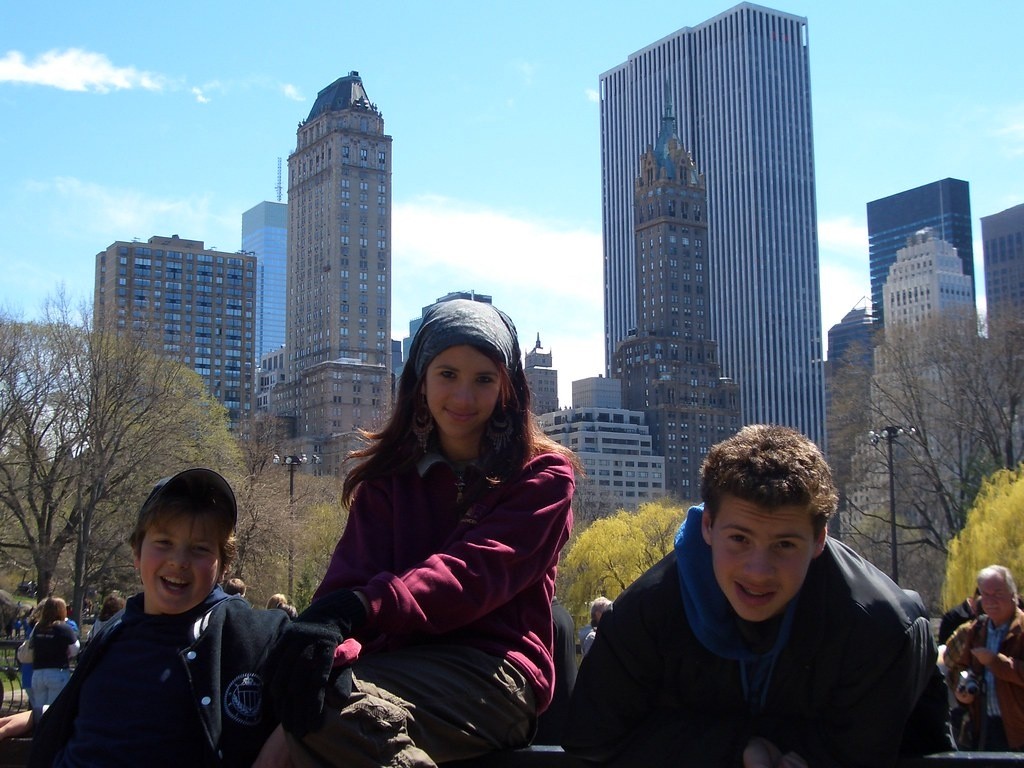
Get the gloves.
[262,589,367,740]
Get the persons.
[558,421,941,767]
[2,465,355,768]
[249,290,587,768]
[933,562,1024,768]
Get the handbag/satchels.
[17,622,39,663]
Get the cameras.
[956,670,980,694]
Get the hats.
[139,468,238,538]
